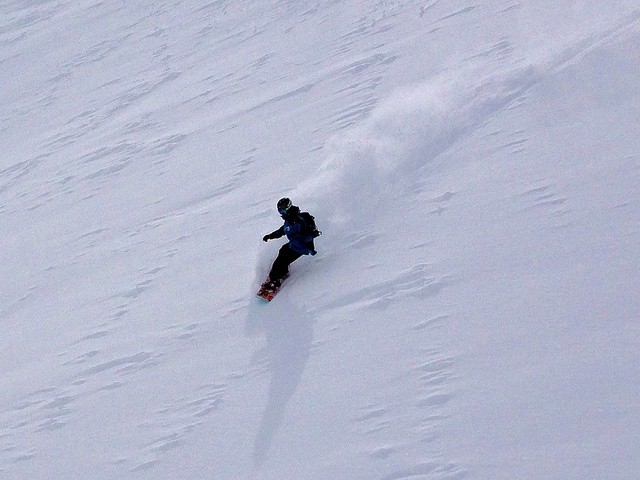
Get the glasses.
[279,210,286,214]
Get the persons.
[262,198,319,290]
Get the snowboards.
[256,269,289,302]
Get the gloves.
[263,235,269,241]
[309,249,317,255]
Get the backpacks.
[301,212,322,238]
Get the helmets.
[277,198,292,212]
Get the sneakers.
[281,274,288,280]
[265,279,281,292]
